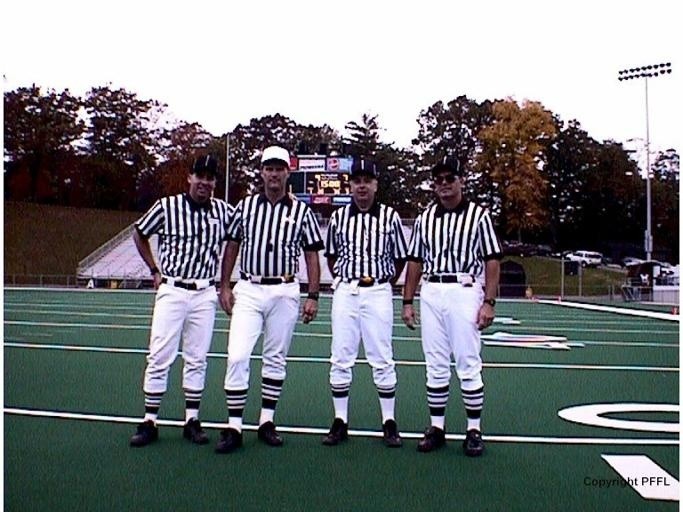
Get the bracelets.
[150,267,159,275]
[403,299,413,306]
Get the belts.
[160,276,215,292]
[239,271,298,286]
[423,275,474,282]
[340,278,387,287]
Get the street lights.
[616,62,672,262]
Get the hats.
[432,155,463,176]
[348,160,377,178]
[260,146,290,168]
[189,154,218,177]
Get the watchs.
[484,298,496,306]
[308,291,319,300]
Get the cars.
[494,235,678,286]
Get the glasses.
[432,173,459,185]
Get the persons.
[401,156,504,456]
[213,145,325,454]
[321,158,407,447]
[130,154,236,447]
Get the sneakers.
[321,418,349,447]
[382,419,403,448]
[214,428,243,453]
[417,426,447,452]
[182,417,210,445]
[129,420,160,448]
[257,421,284,448]
[462,428,485,456]
